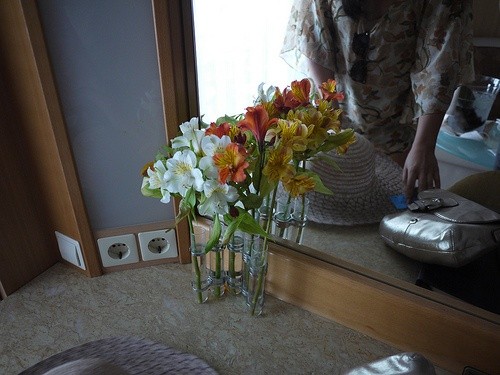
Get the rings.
[432,169,439,174]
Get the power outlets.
[138,229,178,262]
[97,234,140,268]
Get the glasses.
[350,31,369,84]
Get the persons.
[279,0,477,205]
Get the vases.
[255,196,308,244]
[190,223,268,317]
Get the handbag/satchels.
[344,352,435,375]
[378,188,500,269]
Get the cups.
[441,76,500,135]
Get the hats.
[263,128,407,224]
[18,337,218,375]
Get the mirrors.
[192,0,500,324]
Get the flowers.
[140,104,333,317]
[255,79,356,243]
